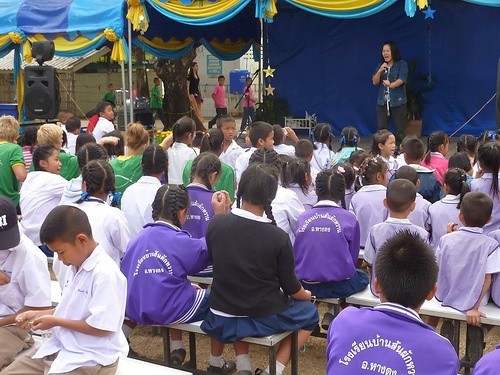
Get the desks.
[118,107,163,130]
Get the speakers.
[23,66,60,119]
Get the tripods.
[235,73,259,142]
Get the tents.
[0,0,500,137]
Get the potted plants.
[405,60,427,138]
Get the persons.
[207,75,227,129]
[149,77,166,126]
[0,100,500,375]
[239,77,257,130]
[372,40,408,145]
[128,81,137,98]
[185,61,205,123]
[101,83,117,106]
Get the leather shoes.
[170,348,186,367]
[207,360,236,375]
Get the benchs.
[14,247,499,375]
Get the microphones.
[254,68,260,75]
